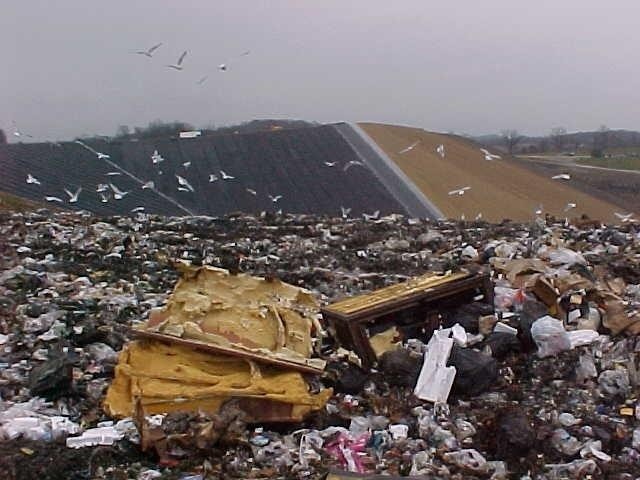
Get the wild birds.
[338,204,382,222]
[11,119,33,140]
[128,41,164,58]
[562,201,577,212]
[551,172,572,183]
[320,158,365,172]
[536,202,544,216]
[612,211,635,224]
[165,50,188,71]
[395,139,504,199]
[24,150,286,215]
